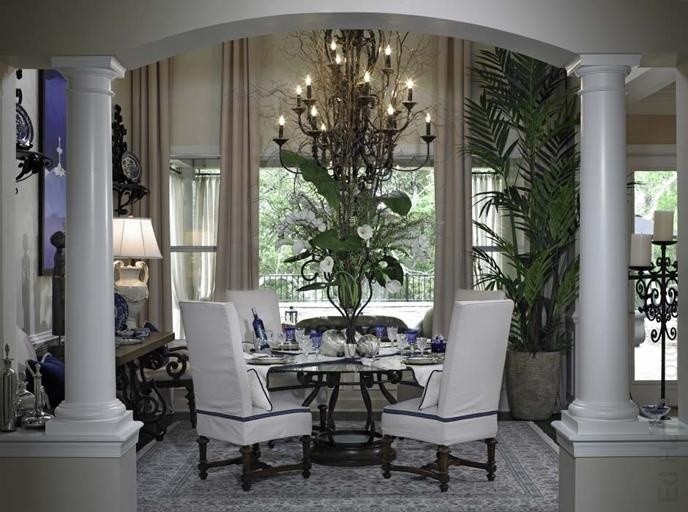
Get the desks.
[46,332,174,441]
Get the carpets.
[135,418,560,511]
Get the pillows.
[25,349,73,424]
[247,369,273,412]
[418,368,441,409]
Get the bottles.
[251,308,269,349]
[285,305,298,326]
[0,342,18,431]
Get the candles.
[630,230,652,266]
[652,211,674,239]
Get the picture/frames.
[37,67,67,275]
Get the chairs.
[225,288,284,449]
[179,299,313,491]
[143,321,197,429]
[377,300,516,491]
[451,288,505,301]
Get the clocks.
[14,70,54,195]
[112,104,151,215]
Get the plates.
[120,151,142,182]
[402,356,433,365]
[244,356,289,365]
[16,103,34,146]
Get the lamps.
[272,28,436,217]
[112,216,165,329]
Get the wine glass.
[374,324,429,358]
[277,327,322,362]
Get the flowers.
[280,149,435,309]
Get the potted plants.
[450,54,647,420]
